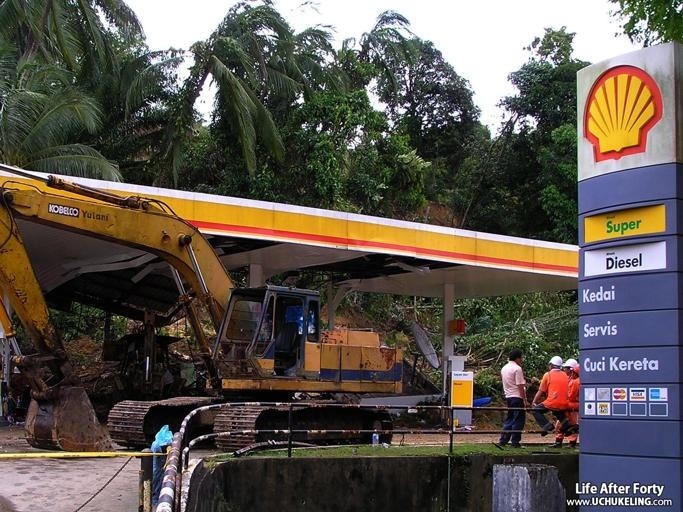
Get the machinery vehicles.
[1,160,414,457]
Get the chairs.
[266,324,299,371]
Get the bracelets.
[532,400,537,403]
[522,397,527,399]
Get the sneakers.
[549,441,562,448]
[512,443,525,449]
[558,418,570,432]
[541,423,555,437]
[565,441,577,449]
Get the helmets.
[570,363,581,375]
[562,358,577,369]
[548,355,562,367]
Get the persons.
[498,349,528,448]
[532,358,577,447]
[531,356,569,436]
[567,365,579,402]
